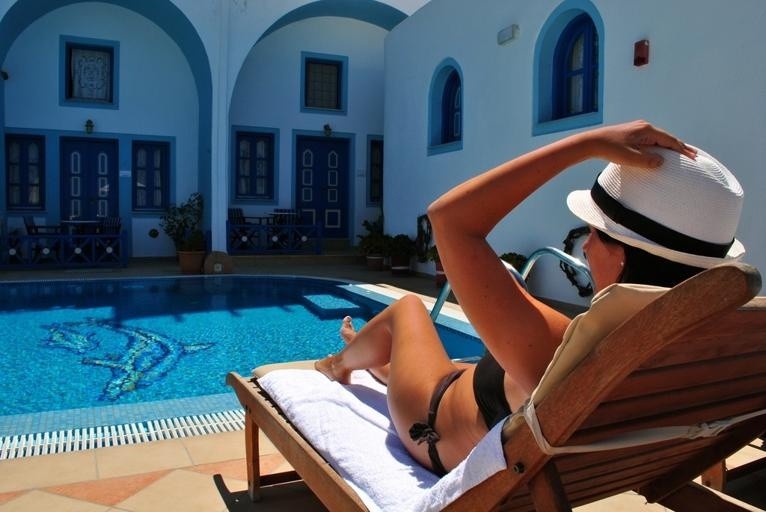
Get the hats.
[566,142,746,269]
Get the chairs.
[22,214,66,260]
[264,208,303,249]
[224,262,766,512]
[228,207,260,251]
[75,215,121,264]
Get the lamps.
[323,124,331,136]
[85,119,94,134]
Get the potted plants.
[356,216,385,271]
[373,233,409,275]
[159,191,206,274]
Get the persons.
[315,118,746,476]
